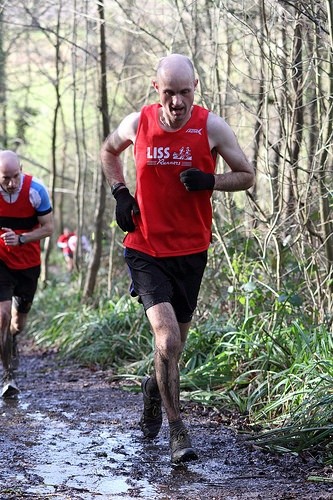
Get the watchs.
[19,234,25,244]
[111,183,125,195]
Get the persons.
[0,150,55,397]
[68,229,90,272]
[57,227,76,272]
[101,54,255,465]
